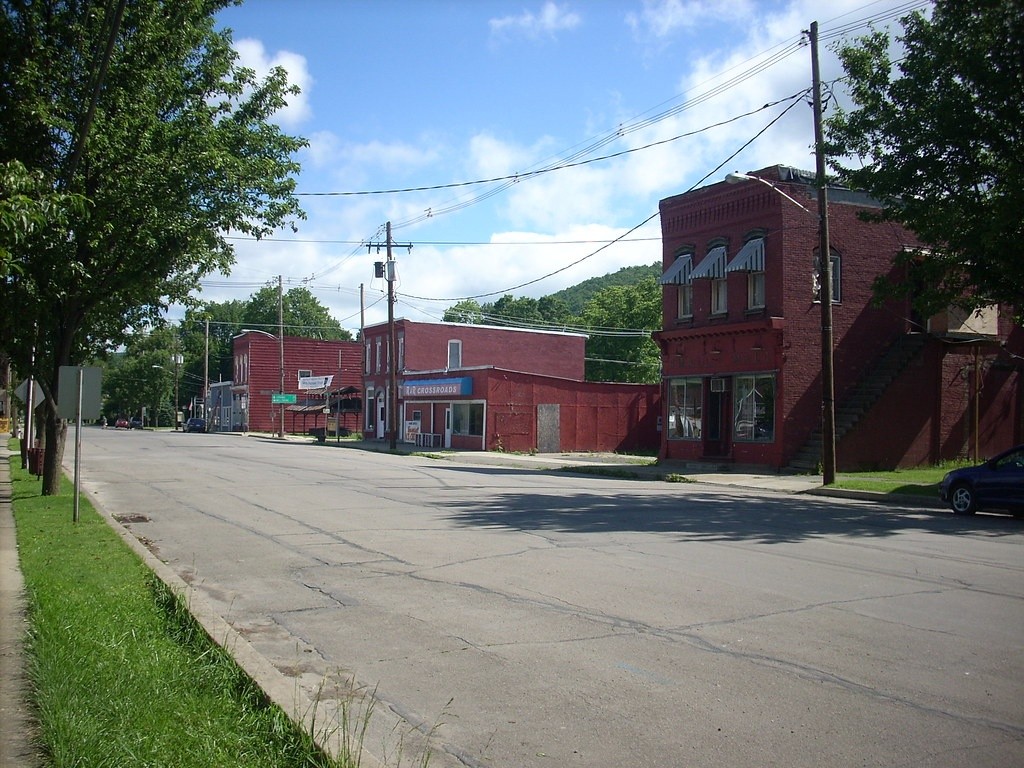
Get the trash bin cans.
[27,448,46,476]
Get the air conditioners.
[711,378,732,392]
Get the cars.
[114,417,130,434]
[938,444,1024,517]
[181,412,206,438]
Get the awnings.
[402,376,473,396]
[724,238,765,273]
[688,247,726,281]
[658,255,692,285]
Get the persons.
[101,416,107,429]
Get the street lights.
[152,365,179,430]
[240,329,285,438]
[724,170,836,484]
